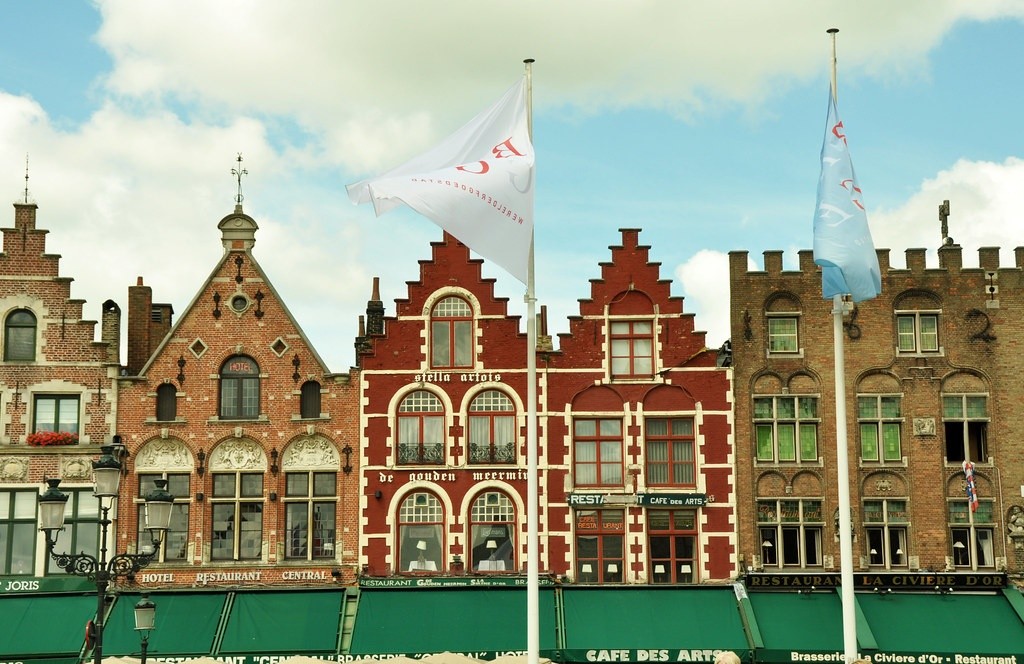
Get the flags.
[814,68,885,304]
[961,462,983,511]
[344,70,534,295]
